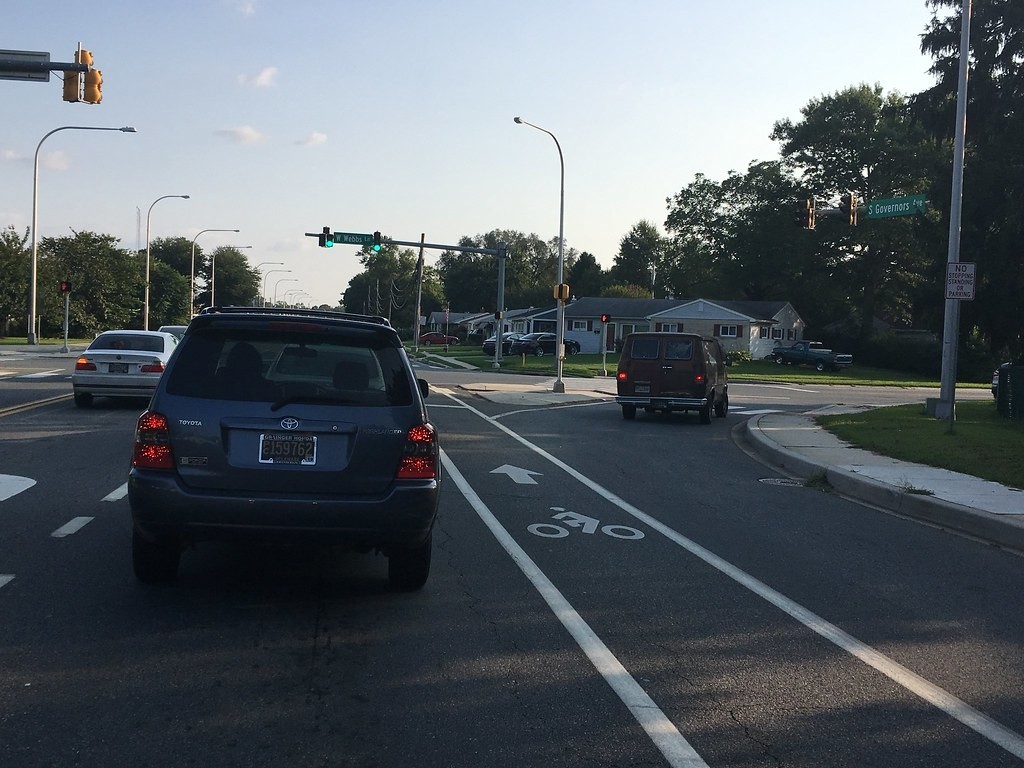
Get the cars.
[418,332,460,345]
[158,323,190,339]
[72,329,179,408]
[512,332,581,357]
[482,331,528,356]
[991,362,1013,400]
[263,341,391,396]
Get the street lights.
[513,115,566,394]
[190,229,241,321]
[253,261,315,310]
[209,246,253,308]
[143,195,191,331]
[25,125,138,345]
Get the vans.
[614,332,733,424]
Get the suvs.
[127,303,444,589]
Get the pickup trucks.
[771,339,855,372]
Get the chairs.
[210,345,277,403]
[330,357,386,405]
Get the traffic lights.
[796,198,812,229]
[839,193,855,224]
[323,226,329,235]
[318,234,325,246]
[373,232,381,251]
[495,312,502,319]
[326,234,333,247]
[61,282,71,292]
[600,313,610,323]
